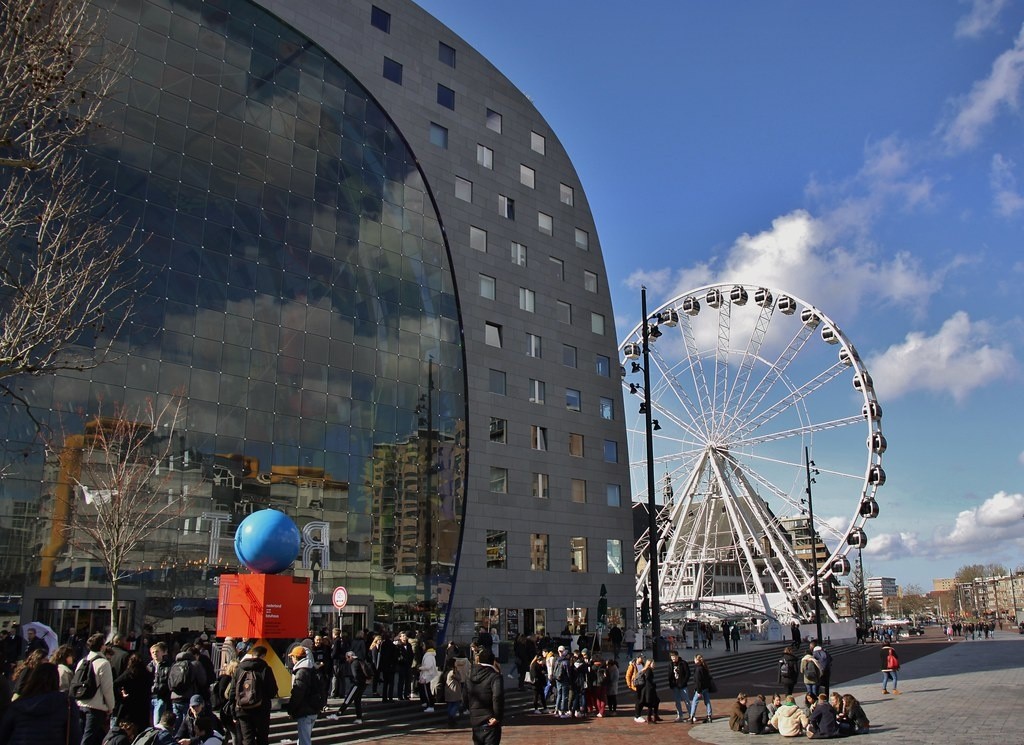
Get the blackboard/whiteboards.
[584,631,601,656]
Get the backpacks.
[555,657,568,683]
[634,668,647,688]
[167,659,195,693]
[131,727,168,745]
[67,651,106,700]
[236,665,267,710]
[593,664,610,686]
[804,659,818,681]
[780,657,795,677]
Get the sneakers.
[633,716,645,723]
[420,702,427,707]
[689,716,697,722]
[353,718,362,724]
[424,707,434,713]
[508,674,514,679]
[673,715,683,722]
[326,713,338,720]
[532,708,618,719]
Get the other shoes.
[685,716,693,723]
[654,714,663,721]
[702,717,712,723]
[646,716,657,724]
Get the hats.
[557,645,565,653]
[189,694,203,707]
[288,646,306,659]
[635,652,646,659]
[346,651,358,659]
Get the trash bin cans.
[652,635,674,662]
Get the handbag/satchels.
[709,674,717,693]
[887,647,898,669]
[524,671,535,684]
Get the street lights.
[630,287,662,661]
[800,446,821,644]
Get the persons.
[879,639,903,695]
[1,623,872,745]
[871,614,1024,647]
[373,598,446,623]
